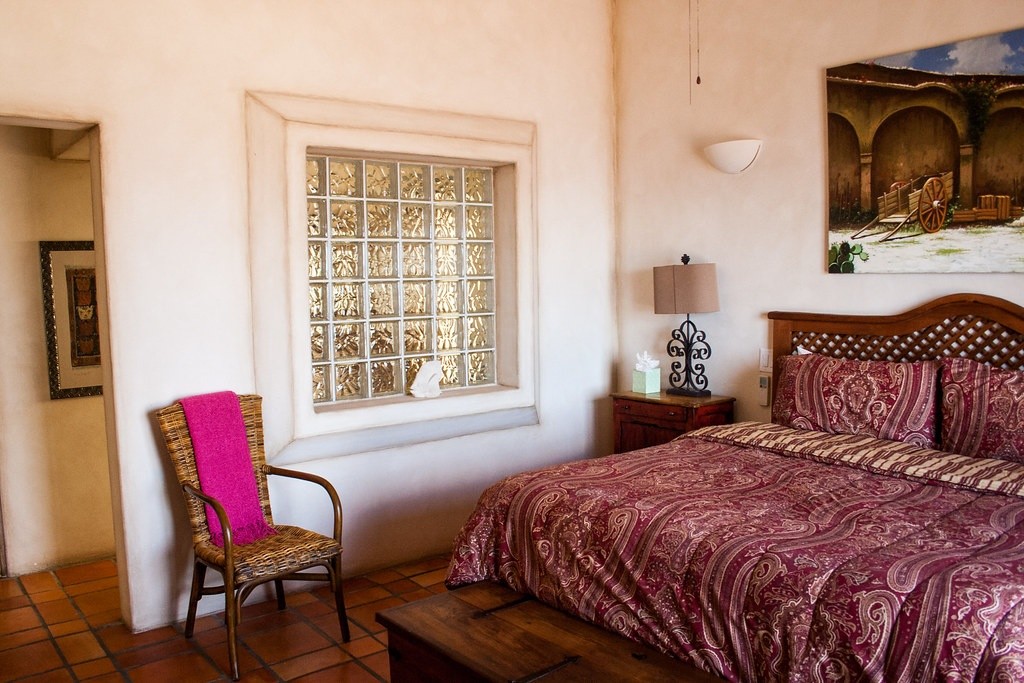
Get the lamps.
[702,139,765,175]
[653,254,720,398]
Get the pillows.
[934,356,1024,462]
[773,354,947,449]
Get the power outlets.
[759,348,773,372]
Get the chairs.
[155,391,350,681]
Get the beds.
[442,293,1024,683]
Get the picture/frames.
[39,240,103,400]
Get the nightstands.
[608,389,737,455]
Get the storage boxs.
[633,368,661,394]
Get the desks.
[373,580,731,683]
